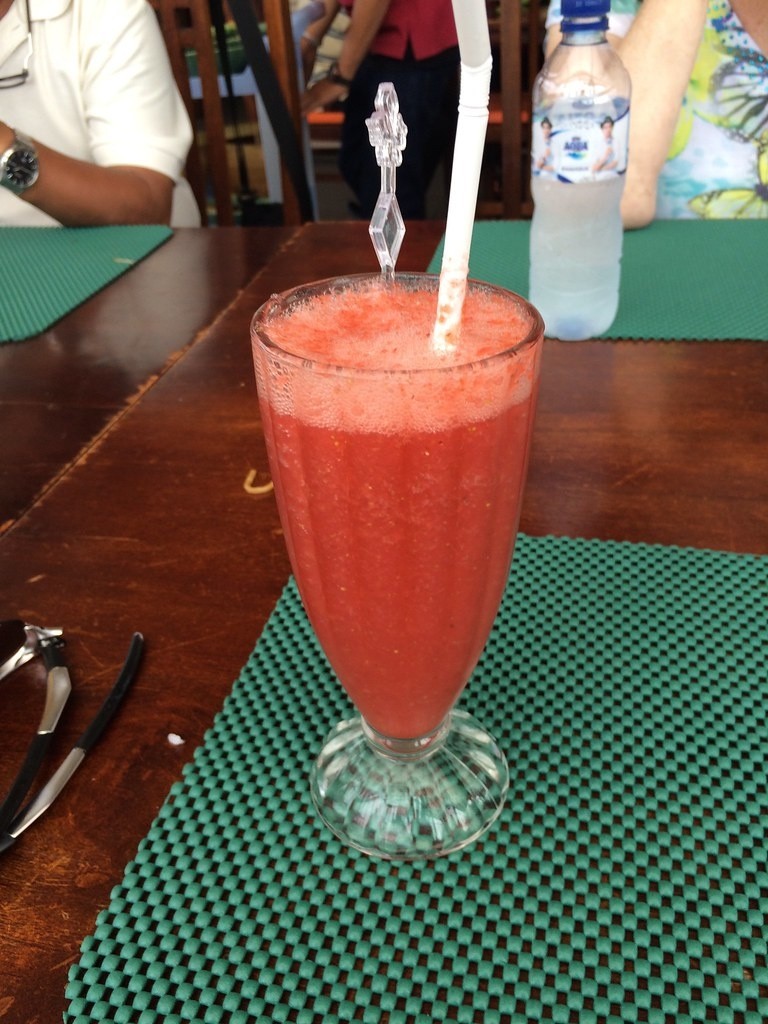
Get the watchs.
[326,63,354,86]
[0,127,40,196]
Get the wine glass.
[250,272,542,863]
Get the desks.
[0,219,308,533]
[0,222,768,1024]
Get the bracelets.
[303,32,320,48]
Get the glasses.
[0,618,148,854]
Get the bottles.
[532,1,631,341]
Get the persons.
[591,116,620,178]
[535,117,564,179]
[296,0,457,224]
[0,0,201,233]
[41,231,208,452]
[544,0,768,231]
